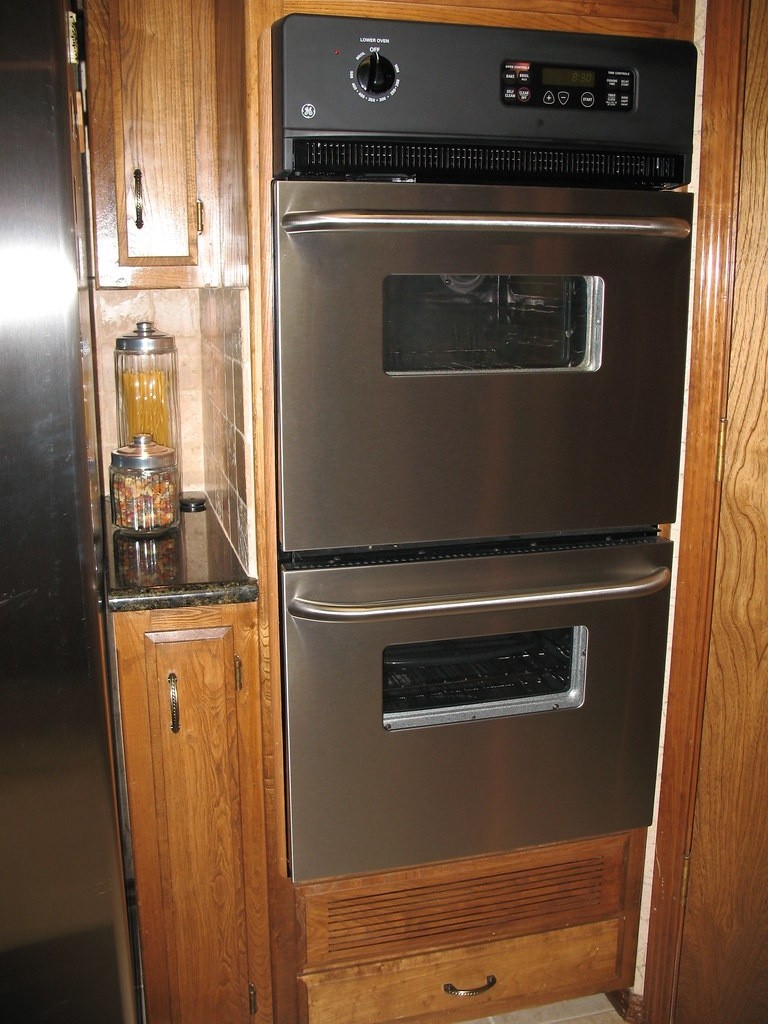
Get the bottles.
[108,432,181,536]
[112,320,182,444]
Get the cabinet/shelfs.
[286,824,649,1023]
[60,0,287,1024]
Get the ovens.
[272,14,699,887]
